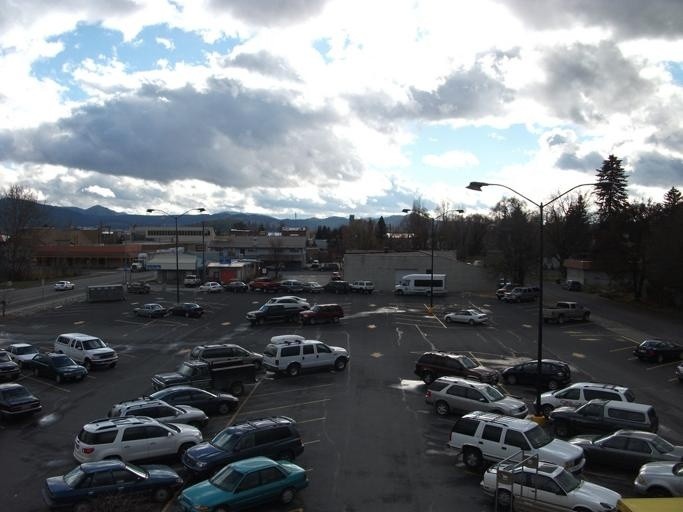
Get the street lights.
[400,206,465,307]
[146,206,206,303]
[462,177,616,426]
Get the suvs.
[183,273,200,287]
[444,408,586,476]
[410,349,497,387]
[421,375,529,420]
[261,333,350,377]
[127,281,151,295]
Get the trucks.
[391,273,447,298]
[129,251,183,273]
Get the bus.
[600,497,683,512]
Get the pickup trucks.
[545,396,661,439]
[52,331,119,371]
[543,300,592,325]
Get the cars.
[443,307,489,327]
[500,357,572,391]
[0,382,44,420]
[632,460,683,497]
[570,428,683,471]
[29,351,89,384]
[631,337,682,362]
[5,342,38,365]
[187,342,265,369]
[52,280,75,291]
[675,361,683,382]
[199,260,375,324]
[476,459,624,512]
[132,303,168,318]
[169,301,203,318]
[0,347,21,377]
[530,380,636,419]
[493,276,540,303]
